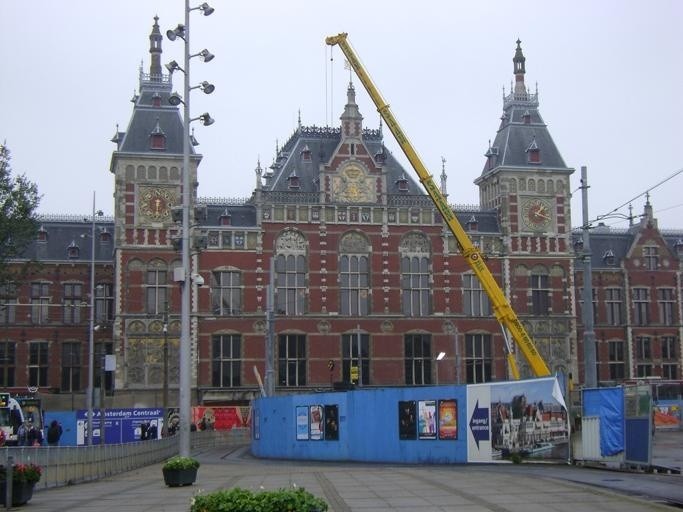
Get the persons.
[140,415,211,441]
[0,425,6,447]
[48,419,63,445]
[17,422,43,446]
[25,407,35,424]
[494,394,544,421]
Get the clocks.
[138,187,175,222]
[521,197,552,230]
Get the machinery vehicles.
[325,32,573,432]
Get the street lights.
[161,299,169,439]
[80,190,110,448]
[165,1,214,461]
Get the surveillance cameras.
[194,276,205,287]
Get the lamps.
[434,352,445,360]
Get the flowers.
[0,463,41,482]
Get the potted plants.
[162,456,199,487]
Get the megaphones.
[165,60,179,74]
[201,112,215,128]
[201,80,215,97]
[167,90,182,107]
[202,47,215,63]
[201,2,214,17]
[165,27,180,42]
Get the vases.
[0,482,34,508]
